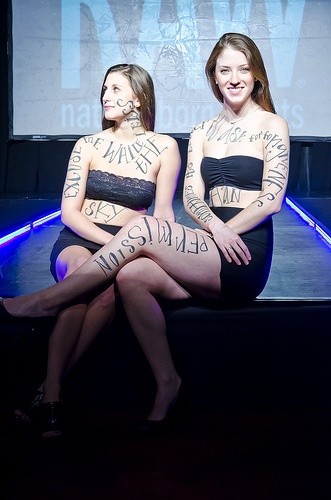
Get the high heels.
[0,296,27,323]
[137,375,185,439]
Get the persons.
[0,33,290,431]
[13,63,181,448]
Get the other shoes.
[47,401,64,426]
[13,389,50,426]
